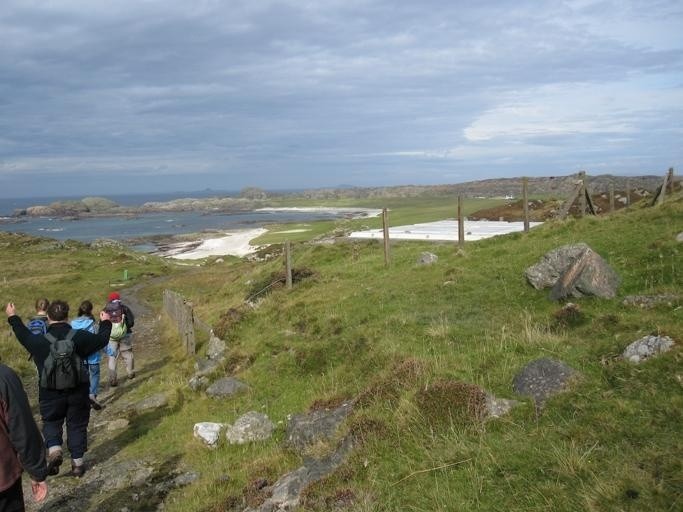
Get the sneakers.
[128,373,133,379]
[89,394,101,410]
[47,450,63,475]
[71,457,84,477]
[110,377,117,386]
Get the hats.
[107,292,121,303]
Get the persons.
[101,291,135,386]
[24,296,49,337]
[0,364,48,511]
[4,299,113,476]
[69,300,125,411]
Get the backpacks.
[104,302,127,341]
[27,316,46,337]
[41,329,84,389]
